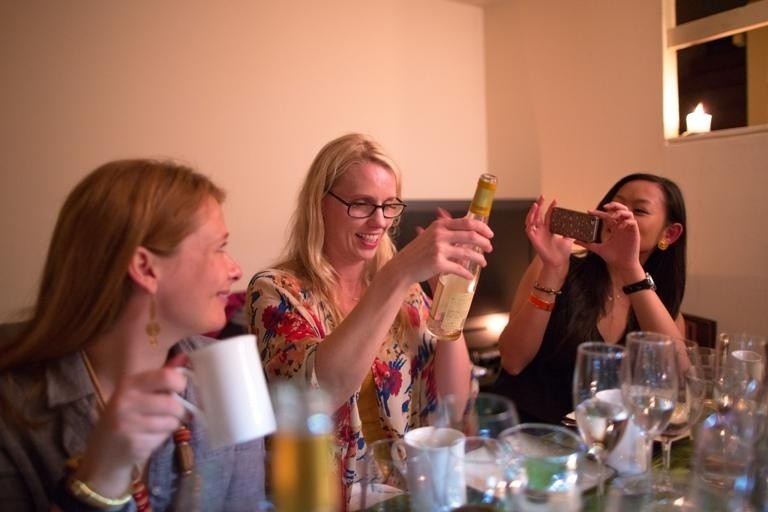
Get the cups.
[681,364,739,446]
[446,437,520,510]
[364,426,466,511]
[504,422,585,510]
[435,394,519,441]
[165,334,276,450]
[685,112,712,132]
[713,332,767,448]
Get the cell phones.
[549,207,599,243]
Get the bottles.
[266,381,342,511]
[426,174,497,342]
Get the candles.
[684,101,712,134]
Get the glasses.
[325,187,408,220]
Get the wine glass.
[622,333,678,506]
[575,341,631,510]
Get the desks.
[352,388,765,512]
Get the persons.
[0,160,268,512]
[244,132,495,511]
[489,172,692,441]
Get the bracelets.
[532,282,562,295]
[527,292,556,312]
[64,455,133,512]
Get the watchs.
[622,272,657,295]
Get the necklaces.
[606,288,624,304]
[80,348,201,512]
